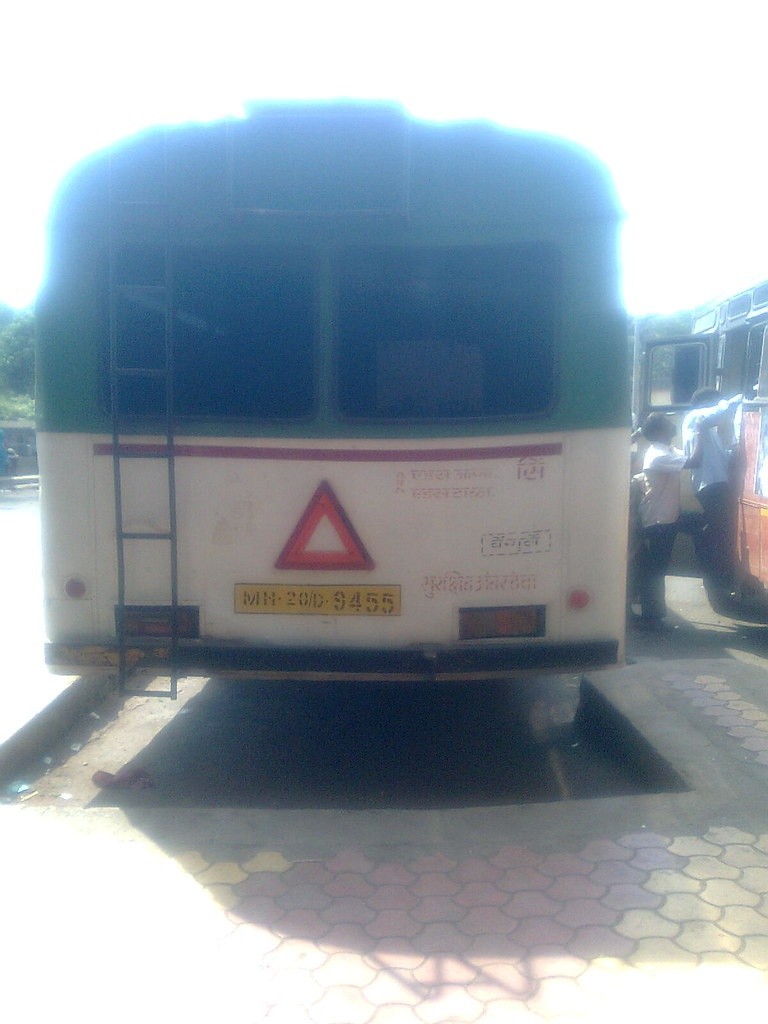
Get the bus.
[30,97,632,750]
[632,280,767,623]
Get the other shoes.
[634,618,679,632]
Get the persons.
[626,388,743,631]
